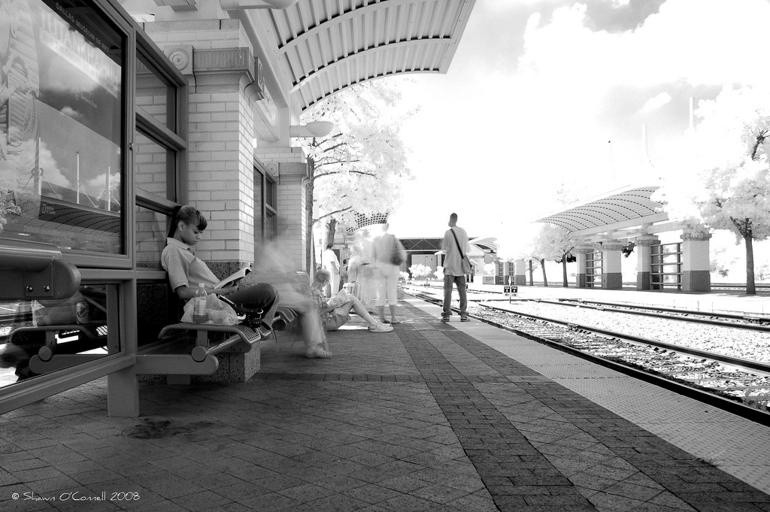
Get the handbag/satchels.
[391,235,402,265]
[462,256,472,273]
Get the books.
[214,261,255,290]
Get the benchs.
[1,306,107,377]
[136,300,303,380]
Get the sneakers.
[441,316,449,322]
[461,318,470,322]
[367,323,394,333]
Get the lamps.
[290,120,335,139]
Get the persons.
[253,218,335,360]
[322,222,406,325]
[160,205,276,329]
[441,213,471,323]
[310,271,394,333]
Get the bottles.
[193,282,209,325]
